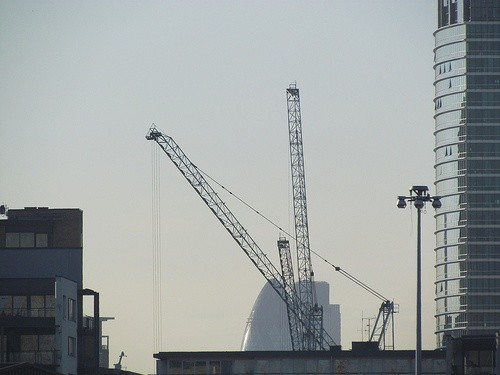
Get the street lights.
[395,185,443,374]
